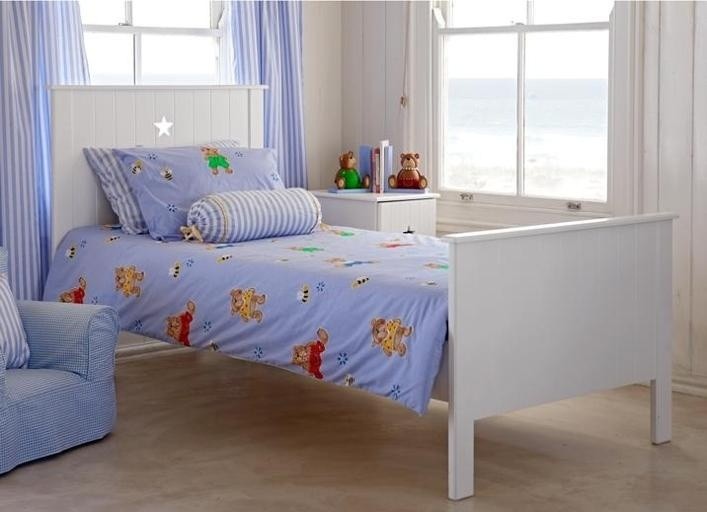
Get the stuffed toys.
[334,151,370,189]
[386,153,428,189]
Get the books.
[370,139,390,194]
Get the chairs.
[0,243,121,480]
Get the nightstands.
[308,189,440,238]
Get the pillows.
[82,138,245,236]
[109,147,285,243]
[181,186,322,243]
[0,273,31,371]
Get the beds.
[48,85,681,501]
